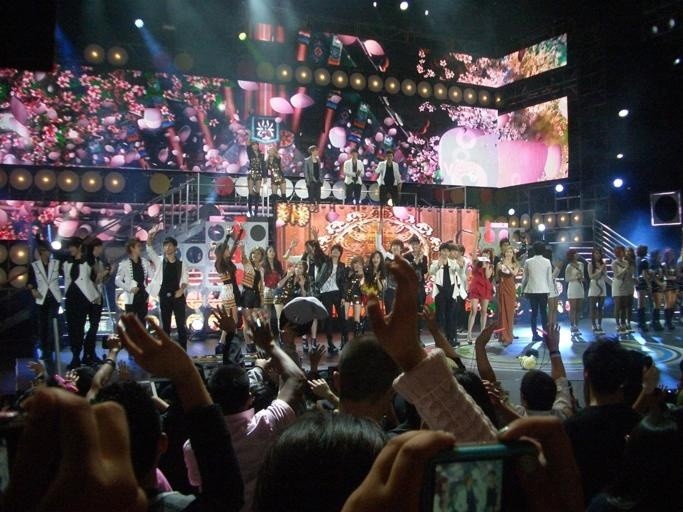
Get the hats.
[279,296,330,337]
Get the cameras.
[422,440,539,512]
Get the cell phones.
[102,335,124,348]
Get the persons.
[304,145,323,203]
[375,150,402,206]
[246,130,263,218]
[344,149,365,205]
[267,148,287,215]
